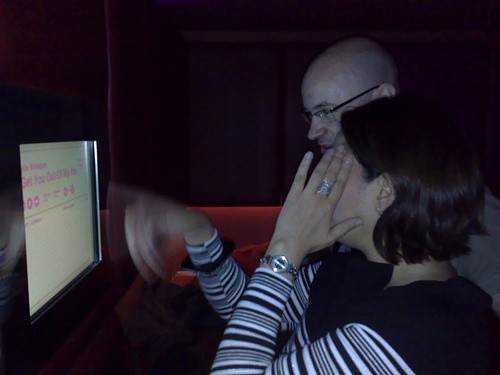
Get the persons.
[124,90,500,375]
[293,41,499,313]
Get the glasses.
[299,86,379,125]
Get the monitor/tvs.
[3,127,107,361]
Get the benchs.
[166,205,284,289]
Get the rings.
[316,177,332,196]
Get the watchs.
[259,254,300,279]
